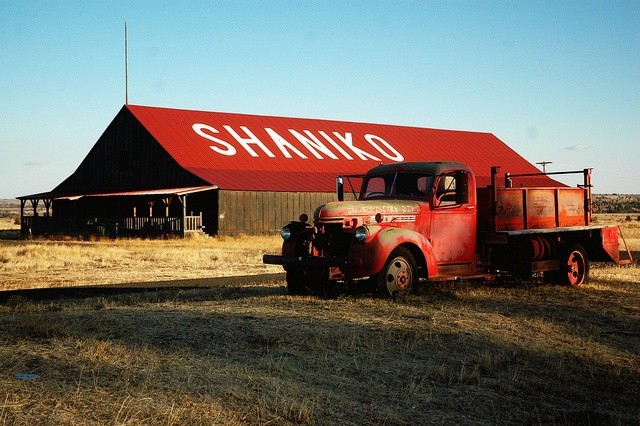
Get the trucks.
[263,159,634,300]
